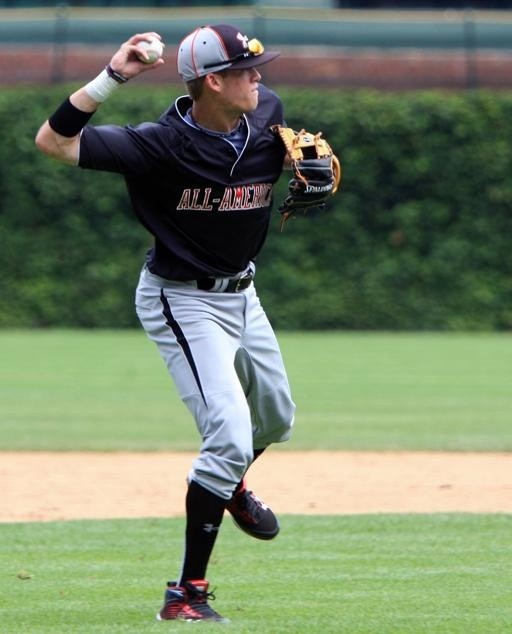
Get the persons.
[35,25,342,624]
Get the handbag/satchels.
[46,95,97,138]
[83,63,129,103]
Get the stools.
[197,276,252,293]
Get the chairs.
[226,480,278,539]
[156,580,229,623]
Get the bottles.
[176,25,280,82]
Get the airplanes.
[273,124,341,222]
